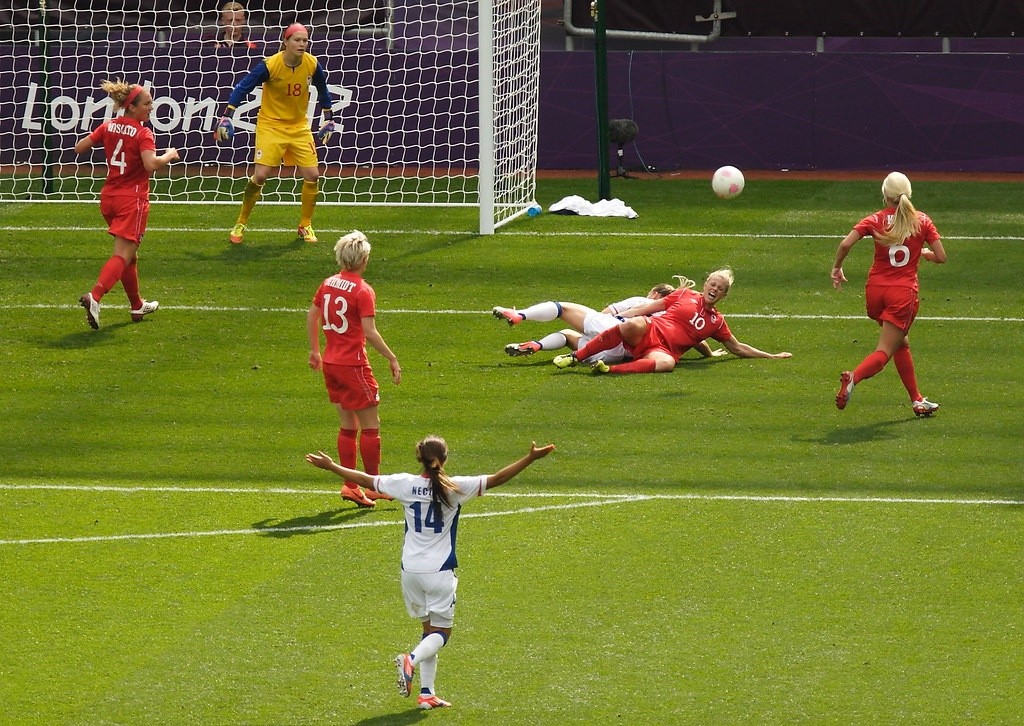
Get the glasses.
[226,18,244,23]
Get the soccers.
[712,166,745,200]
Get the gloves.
[318,119,335,144]
[213,117,235,142]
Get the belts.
[619,317,625,323]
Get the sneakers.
[131,300,159,322]
[493,305,524,327]
[392,653,415,698]
[366,487,395,501]
[229,223,246,244]
[588,359,611,374]
[503,341,541,357]
[298,225,319,243]
[834,370,854,410]
[912,397,940,417]
[341,483,376,507]
[79,293,100,330]
[417,695,452,710]
[552,351,580,368]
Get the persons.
[217,23,335,242]
[205,2,257,48]
[305,435,556,709]
[831,172,947,416]
[308,231,401,507]
[553,269,792,374]
[492,276,696,364]
[75,77,180,329]
